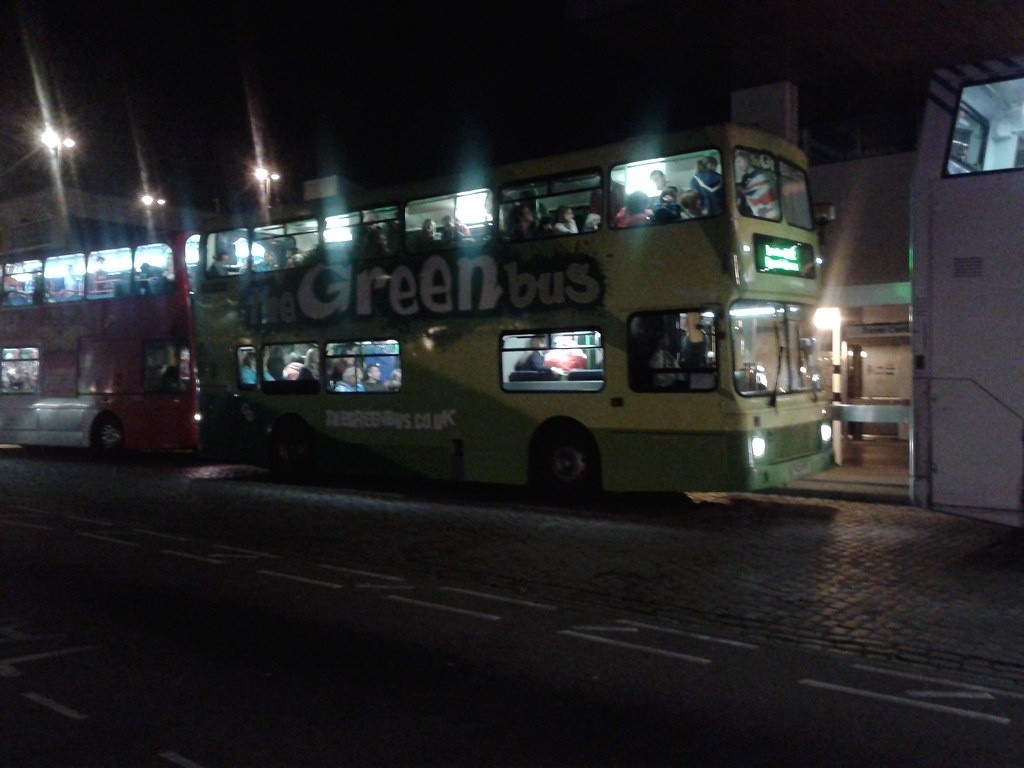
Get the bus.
[1,231,200,456]
[196,123,840,505]
[909,52,1024,534]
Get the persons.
[514,336,604,371]
[142,345,191,394]
[207,249,229,277]
[240,343,403,392]
[241,235,302,275]
[345,213,472,259]
[485,151,781,243]
[0,252,194,306]
[6,367,32,390]
[639,328,718,393]
